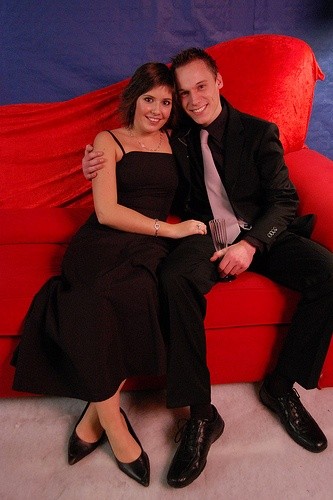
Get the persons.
[82,47,333,488]
[9,62,207,488]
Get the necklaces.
[129,128,163,152]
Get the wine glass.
[208,218,236,283]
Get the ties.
[200,129,241,245]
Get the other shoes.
[68,402,107,465]
[103,407,150,487]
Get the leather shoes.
[167,404,225,489]
[258,382,328,453]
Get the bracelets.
[154,219,160,237]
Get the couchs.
[0,36,333,393]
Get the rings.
[197,225,200,230]
